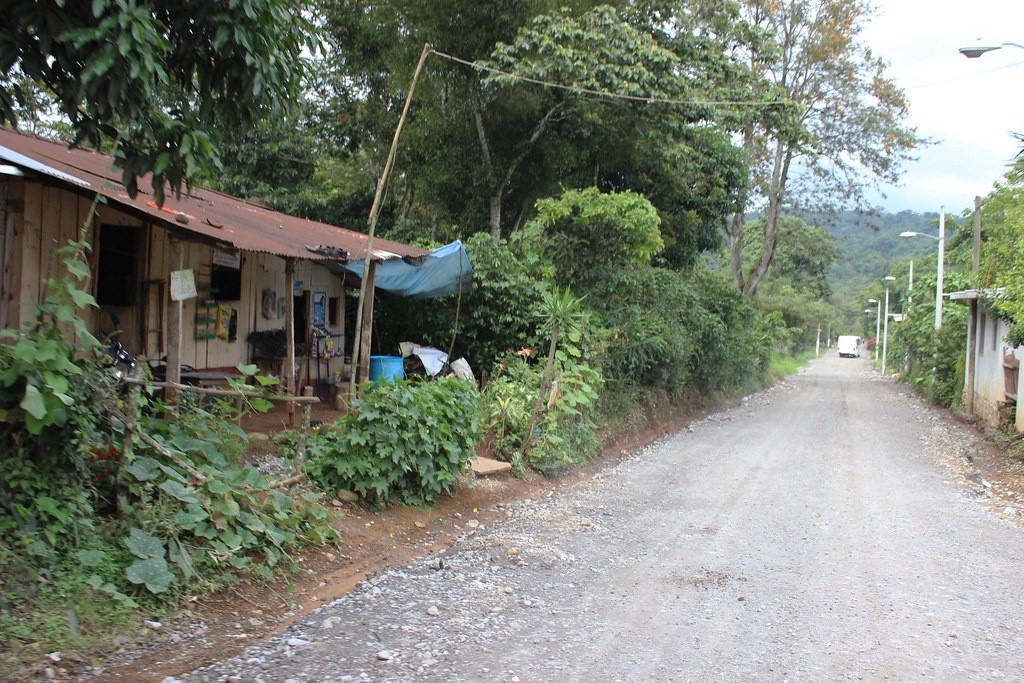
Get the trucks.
[838,335,865,358]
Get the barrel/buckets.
[369,356,404,391]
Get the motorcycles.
[92,312,200,418]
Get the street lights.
[958,40,1024,58]
[898,231,946,382]
[882,277,914,374]
[864,297,882,362]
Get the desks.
[160,371,247,426]
[254,352,309,396]
